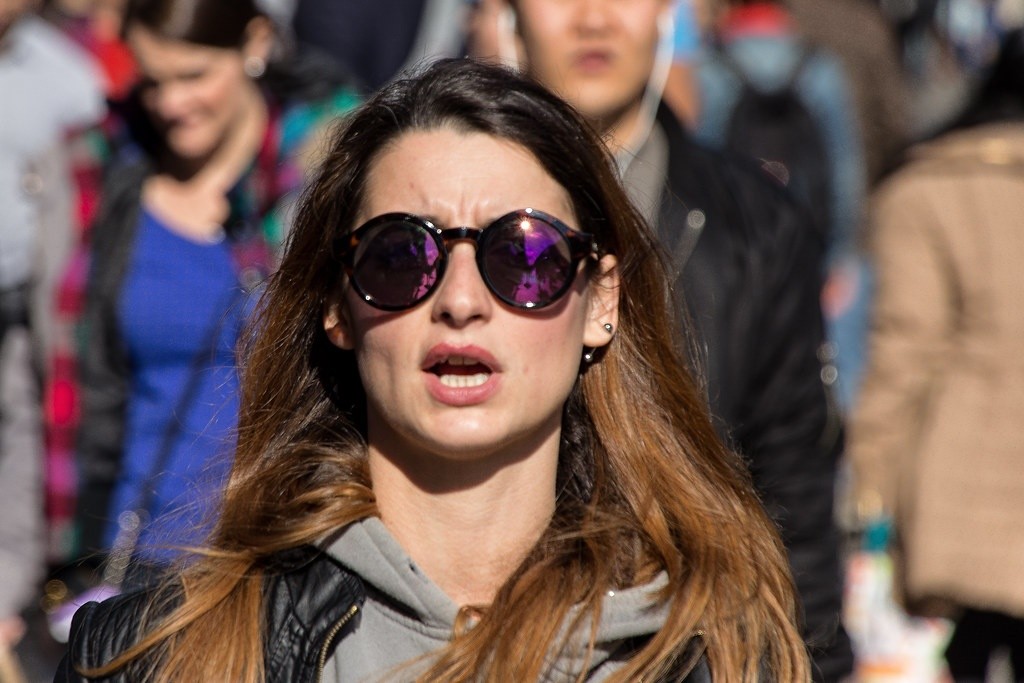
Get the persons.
[1,0,1024,682]
[55,53,812,683]
[827,26,1024,683]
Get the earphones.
[498,11,516,36]
[658,12,677,38]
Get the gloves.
[840,554,955,671]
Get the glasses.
[333,213,599,310]
